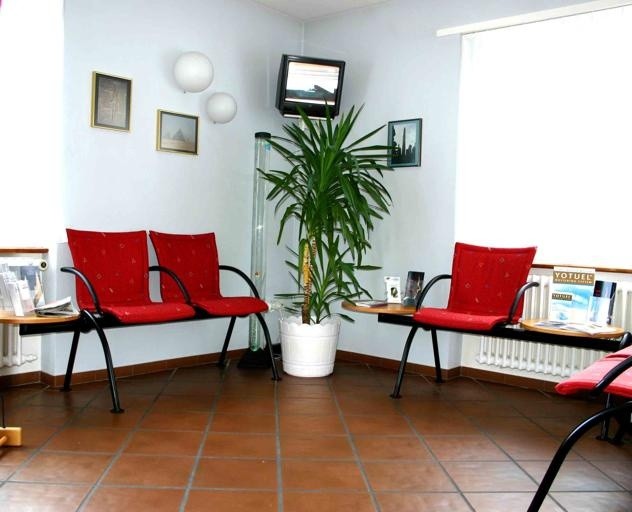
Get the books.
[590,280,617,324]
[547,266,596,322]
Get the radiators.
[475,275,632,377]
[0,257,37,370]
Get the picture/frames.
[91,70,133,133]
[156,109,201,156]
[387,118,422,168]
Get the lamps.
[206,93,237,124]
[174,51,214,93]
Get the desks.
[342,300,424,315]
[0,310,81,324]
[520,318,625,338]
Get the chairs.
[412,242,539,331]
[527,344,632,512]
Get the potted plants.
[255,95,395,378]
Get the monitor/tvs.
[276,55,345,121]
